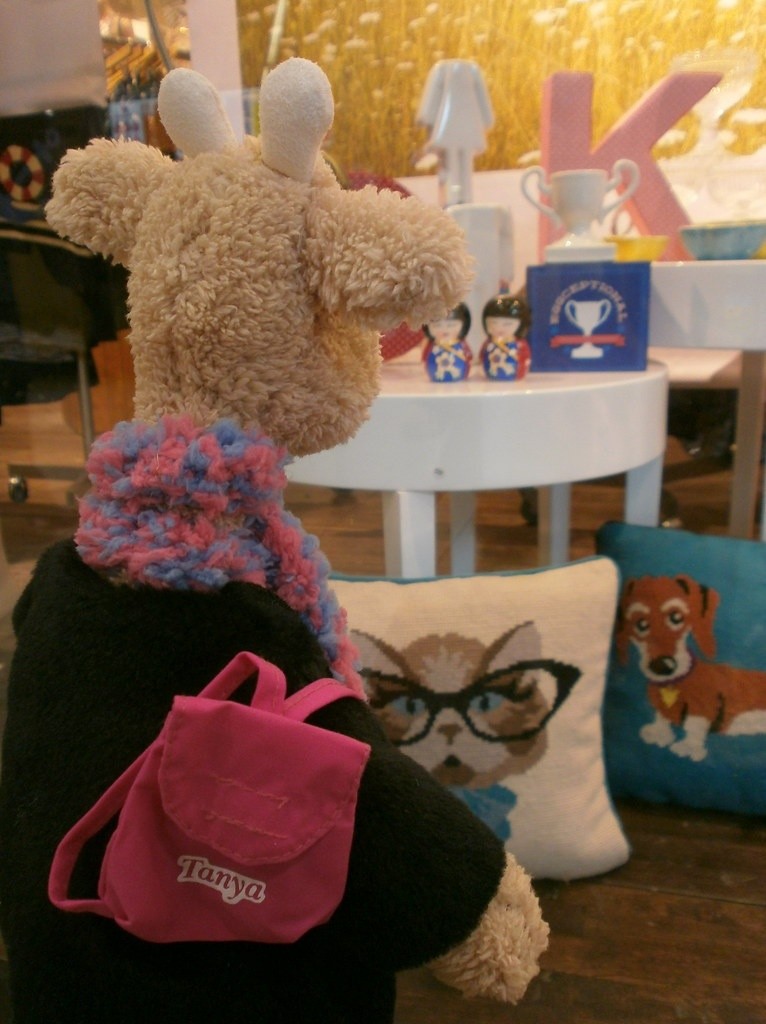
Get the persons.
[422,295,533,384]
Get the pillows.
[596,521,766,816]
[329,556,633,886]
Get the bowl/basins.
[607,234,669,262]
[680,219,766,260]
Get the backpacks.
[47,652,371,938]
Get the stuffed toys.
[0,58,550,1024]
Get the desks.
[281,349,668,580]
[650,258,763,538]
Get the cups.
[520,159,640,261]
